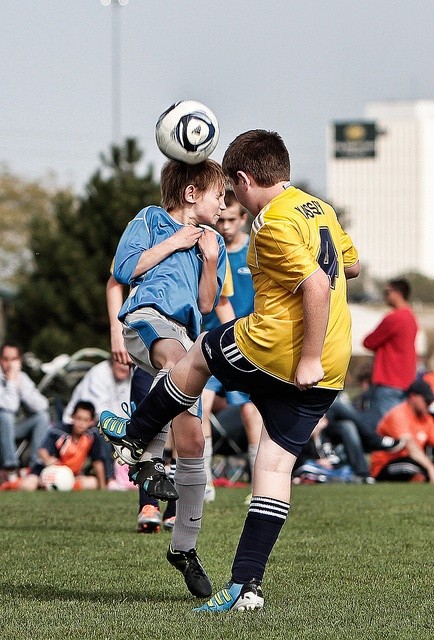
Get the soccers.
[155,99,219,165]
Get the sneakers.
[138,505,162,531]
[189,578,265,612]
[97,402,148,467]
[166,542,212,598]
[127,458,179,502]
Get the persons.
[314,402,402,485]
[111,159,227,596]
[419,354,434,391]
[372,381,434,481]
[106,254,237,536]
[1,402,106,493]
[365,277,419,416]
[214,390,264,456]
[354,374,371,410]
[99,130,360,609]
[63,351,142,491]
[0,343,49,471]
[201,190,264,503]
